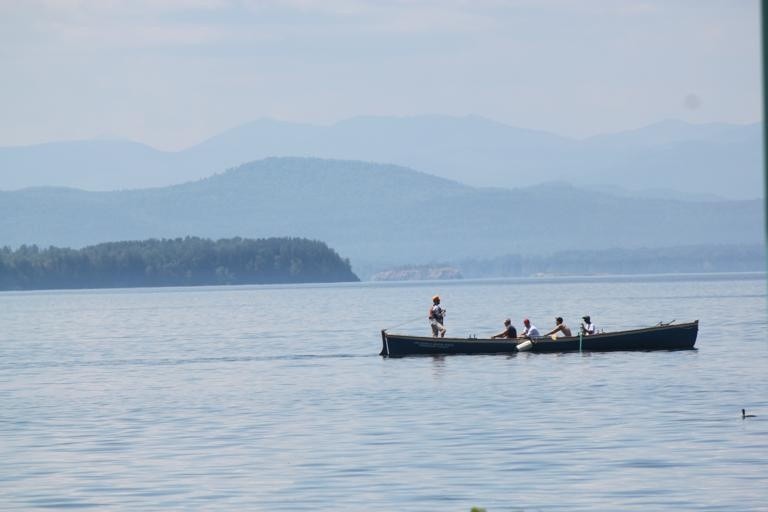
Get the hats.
[523,319,529,324]
[582,315,590,319]
[432,295,441,302]
[555,316,562,321]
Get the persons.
[517,318,540,339]
[543,316,572,337]
[579,315,594,336]
[489,319,517,339]
[427,295,446,339]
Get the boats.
[380,319,699,357]
